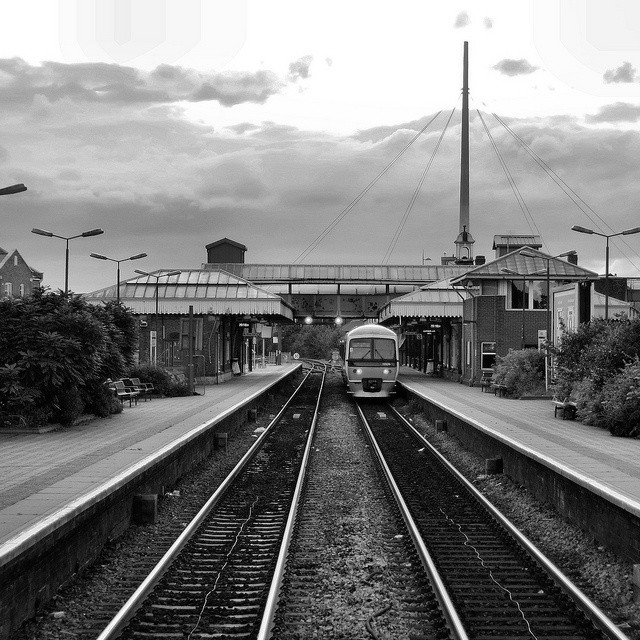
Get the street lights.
[32,228,104,294]
[519,250,577,339]
[90,253,147,304]
[501,268,547,349]
[135,269,181,330]
[571,224,640,320]
[0,184,27,196]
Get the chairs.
[553,381,579,420]
[492,378,508,396]
[113,381,139,407]
[481,378,492,392]
[129,378,154,402]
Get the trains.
[339,324,399,397]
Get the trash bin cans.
[427,359,435,374]
[232,358,240,375]
